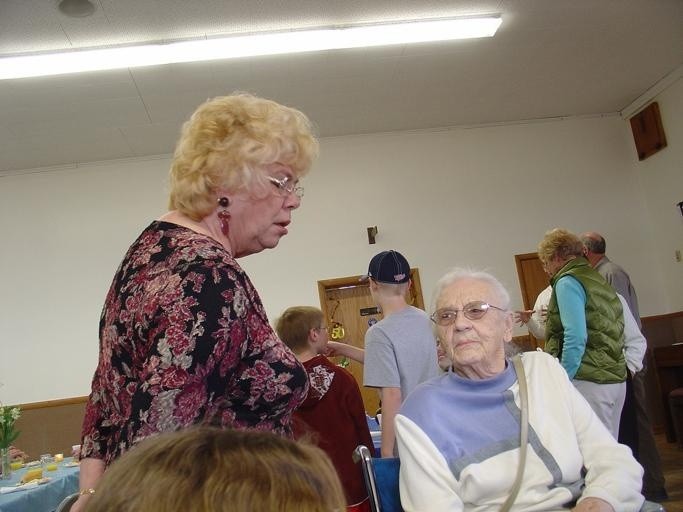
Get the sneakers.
[645,491,668,501]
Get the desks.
[648,341,683,448]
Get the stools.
[667,387,683,448]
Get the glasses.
[429,301,507,326]
[265,175,305,198]
[316,327,331,334]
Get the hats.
[359,250,410,284]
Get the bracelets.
[80,488,95,497]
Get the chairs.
[351,444,404,512]
[55,492,81,512]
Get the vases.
[0,452,10,480]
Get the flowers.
[0,405,23,480]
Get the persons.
[77,427,349,512]
[323,249,439,511]
[514,284,649,463]
[576,233,670,512]
[275,306,375,511]
[71,449,82,461]
[66,91,319,511]
[392,270,664,512]
[536,226,648,445]
[0,446,29,475]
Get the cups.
[40,454,49,464]
[54,450,64,462]
[72,445,81,462]
[10,459,22,471]
